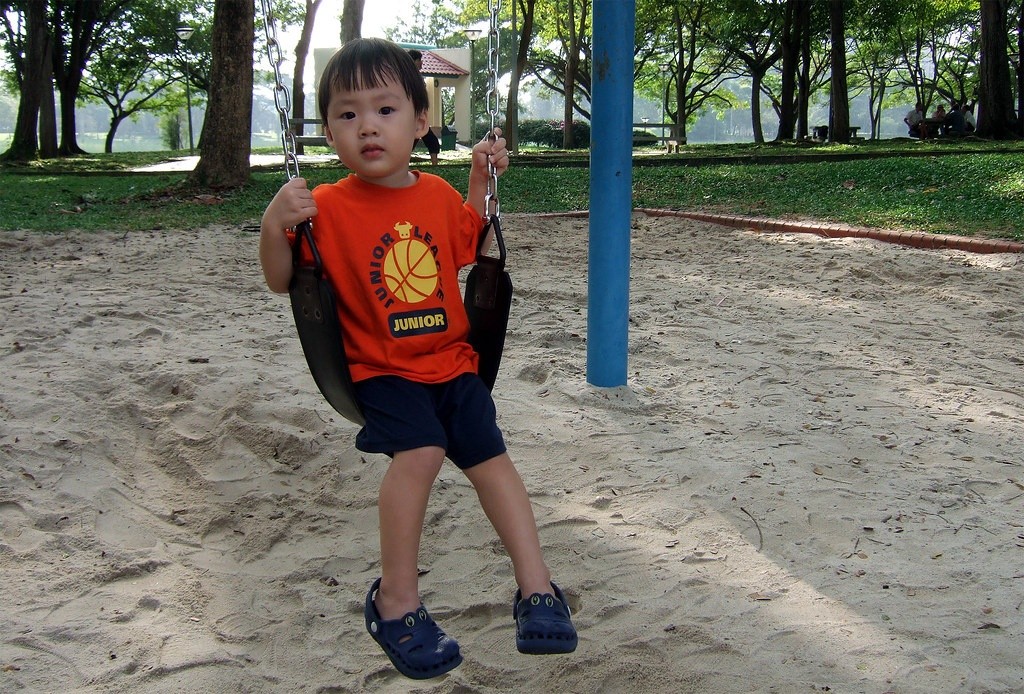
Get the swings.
[261,0,514,426]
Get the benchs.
[286,135,326,142]
[633,137,687,141]
[817,136,864,139]
[949,131,976,135]
[908,131,917,134]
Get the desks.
[285,119,324,152]
[919,120,942,138]
[809,126,861,143]
[633,124,683,153]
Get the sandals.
[364,577,463,680]
[513,581,578,655]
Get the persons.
[260,38,578,681]
[933,100,975,137]
[407,50,440,166]
[904,102,932,139]
